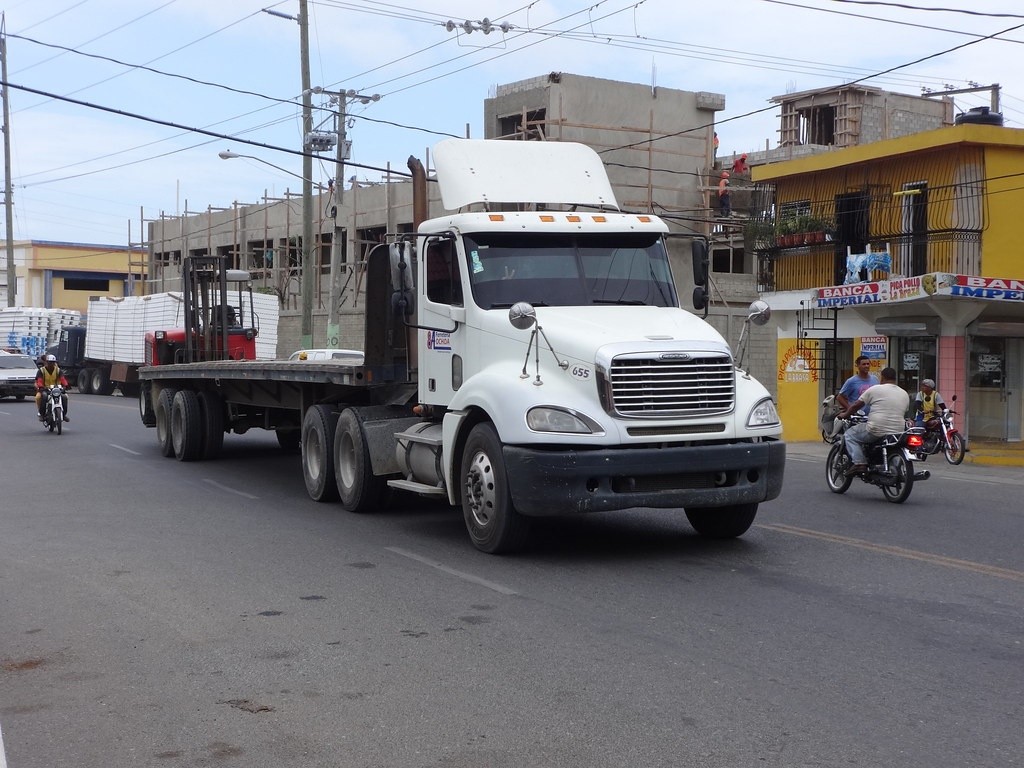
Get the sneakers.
[842,464,866,477]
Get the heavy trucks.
[136,139,786,557]
[45,324,146,398]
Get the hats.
[923,379,935,388]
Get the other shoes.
[37,412,41,416]
[922,456,925,461]
[39,416,44,421]
[917,456,922,459]
[63,415,71,422]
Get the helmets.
[46,355,56,361]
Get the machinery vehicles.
[140,251,260,427]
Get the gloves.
[65,384,72,391]
[38,387,45,393]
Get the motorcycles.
[37,384,72,435]
[825,409,931,504]
[906,394,965,465]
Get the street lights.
[216,151,345,351]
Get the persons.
[837,368,909,475]
[34,355,71,422]
[732,154,750,174]
[908,379,946,429]
[720,171,731,218]
[714,131,720,164]
[838,356,879,420]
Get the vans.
[0,353,40,401]
